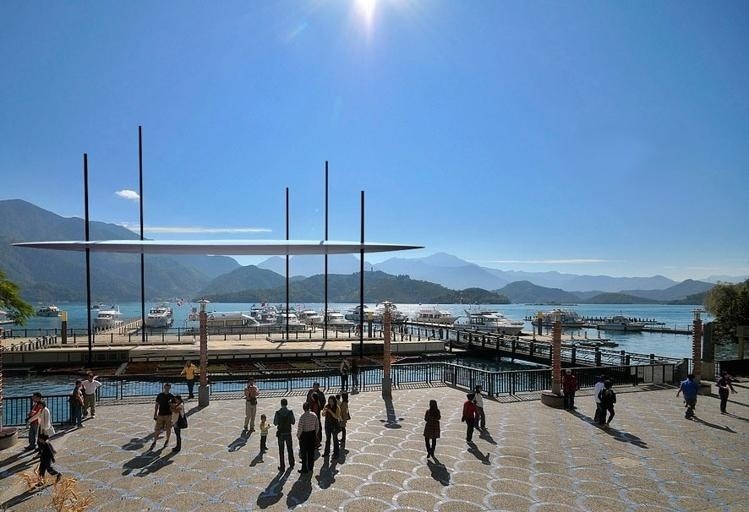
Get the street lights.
[381,301,393,400]
[195,300,211,407]
[0,311,18,450]
[689,310,711,396]
[541,310,566,408]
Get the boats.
[144,304,177,330]
[410,302,461,325]
[451,302,526,336]
[597,314,648,332]
[86,302,106,313]
[185,297,408,336]
[94,308,125,329]
[35,302,65,317]
[530,308,590,328]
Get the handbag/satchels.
[178,413,187,428]
[46,426,55,437]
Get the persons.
[27,400,56,460]
[715,371,738,414]
[152,384,176,448]
[82,373,102,419]
[24,392,42,452]
[35,433,62,487]
[462,394,479,443]
[598,380,617,428]
[260,414,271,453]
[676,374,699,420]
[423,400,441,458]
[473,385,488,429]
[273,355,359,473]
[562,368,578,410]
[71,381,86,428]
[242,378,260,432]
[168,396,187,452]
[181,360,197,399]
[593,374,608,425]
